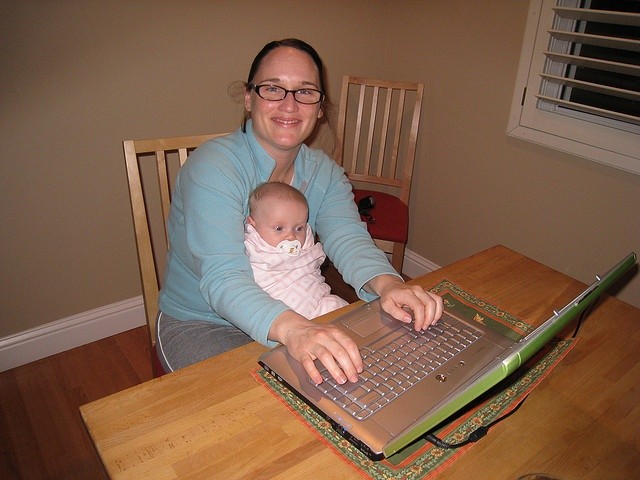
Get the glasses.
[251,83,325,104]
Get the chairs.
[122,133,232,379]
[334,73,425,275]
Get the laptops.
[258,251,637,462]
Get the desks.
[80,245,640,480]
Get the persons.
[154,38,444,385]
[244,181,350,320]
